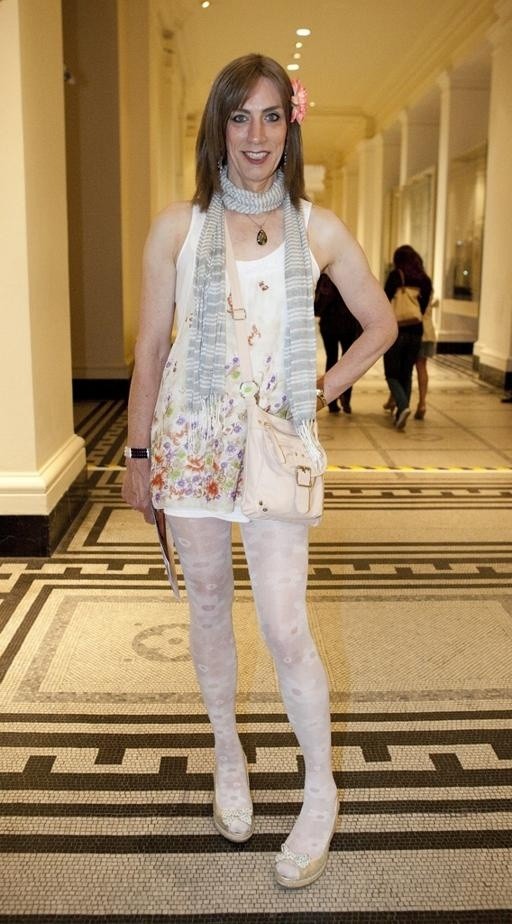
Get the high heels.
[383,398,396,416]
[414,403,426,420]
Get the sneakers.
[394,407,411,429]
[328,399,351,414]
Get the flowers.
[290,76,308,124]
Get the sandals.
[211,753,255,844]
[273,791,340,889]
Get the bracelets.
[122,446,151,459]
[316,375,327,410]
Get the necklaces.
[245,210,274,247]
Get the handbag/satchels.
[239,409,325,524]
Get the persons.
[315,269,362,413]
[381,262,437,420]
[120,51,404,891]
[380,245,431,429]
